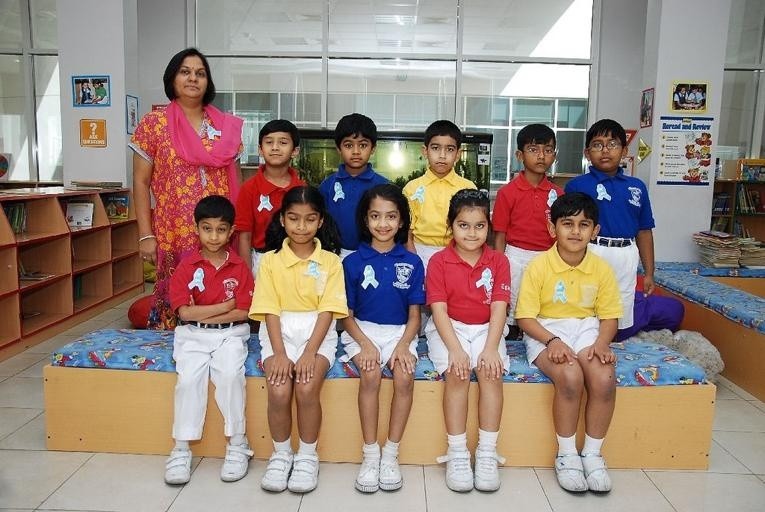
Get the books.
[104,195,129,220]
[693,183,765,269]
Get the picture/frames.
[639,88,654,128]
[667,81,710,114]
[71,74,111,107]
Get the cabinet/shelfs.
[0,185,145,350]
[711,179,764,250]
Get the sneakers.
[436,446,474,492]
[354,444,380,493]
[287,451,320,493]
[220,436,254,482]
[555,455,589,492]
[475,445,506,492]
[261,448,294,492]
[580,453,612,492]
[164,446,192,485]
[379,445,403,491]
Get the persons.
[748,166,755,180]
[92,79,108,105]
[672,86,705,110]
[79,81,93,104]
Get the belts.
[589,235,636,247]
[180,319,248,329]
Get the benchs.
[636,260,764,299]
[42,327,717,471]
[650,270,764,403]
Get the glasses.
[588,139,623,152]
[523,148,557,156]
